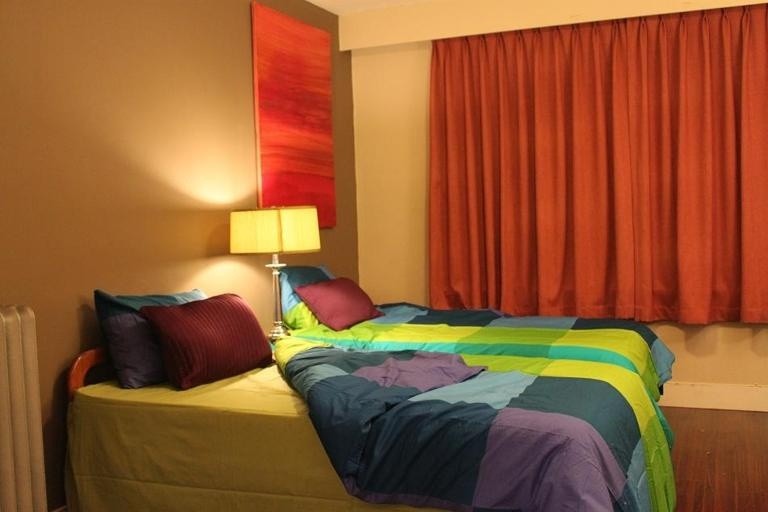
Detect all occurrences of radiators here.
[0,304,48,512]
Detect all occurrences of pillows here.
[138,292,277,389]
[94,288,209,389]
[294,276,386,331]
[278,265,337,332]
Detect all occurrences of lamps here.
[229,206,321,346]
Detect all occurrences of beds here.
[286,303,675,402]
[64,347,677,512]
